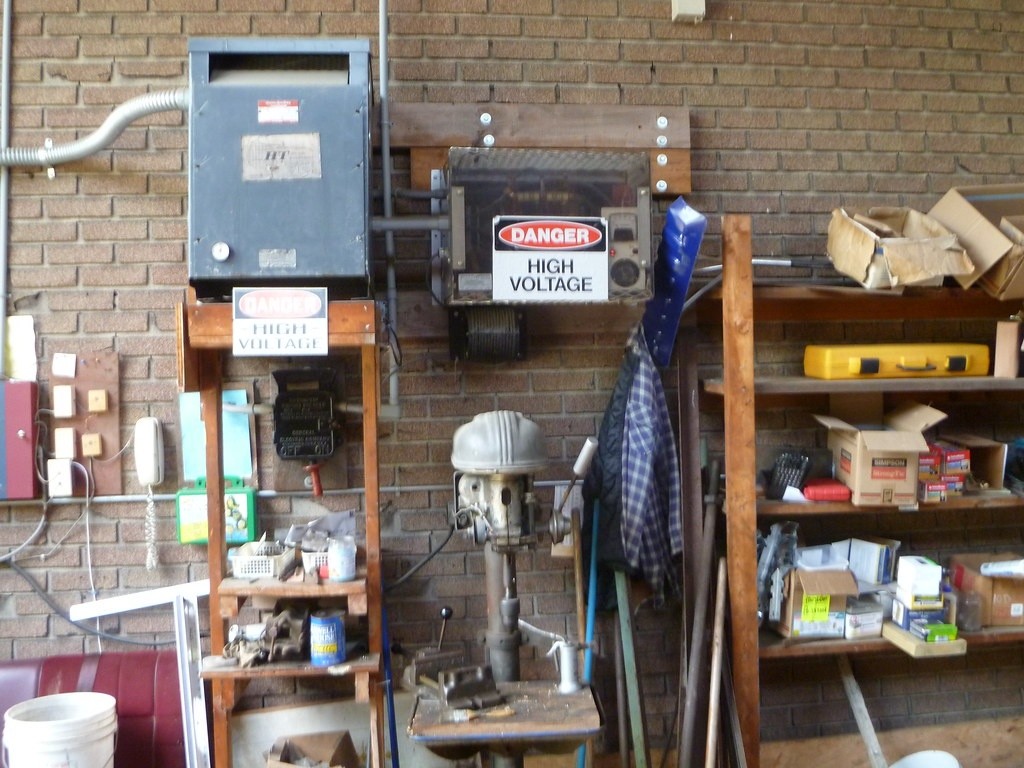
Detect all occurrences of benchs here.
[1,651,187,768]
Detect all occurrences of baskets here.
[300,547,329,575]
[229,540,295,579]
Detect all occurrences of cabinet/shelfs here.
[681,209,1024,768]
[177,285,387,768]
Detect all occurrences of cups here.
[329,537,356,581]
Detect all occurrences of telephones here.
[135,415,166,488]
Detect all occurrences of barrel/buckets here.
[1,692,118,768]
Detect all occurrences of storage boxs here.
[765,535,1023,657]
[809,391,948,506]
[267,729,359,768]
[827,181,1023,302]
